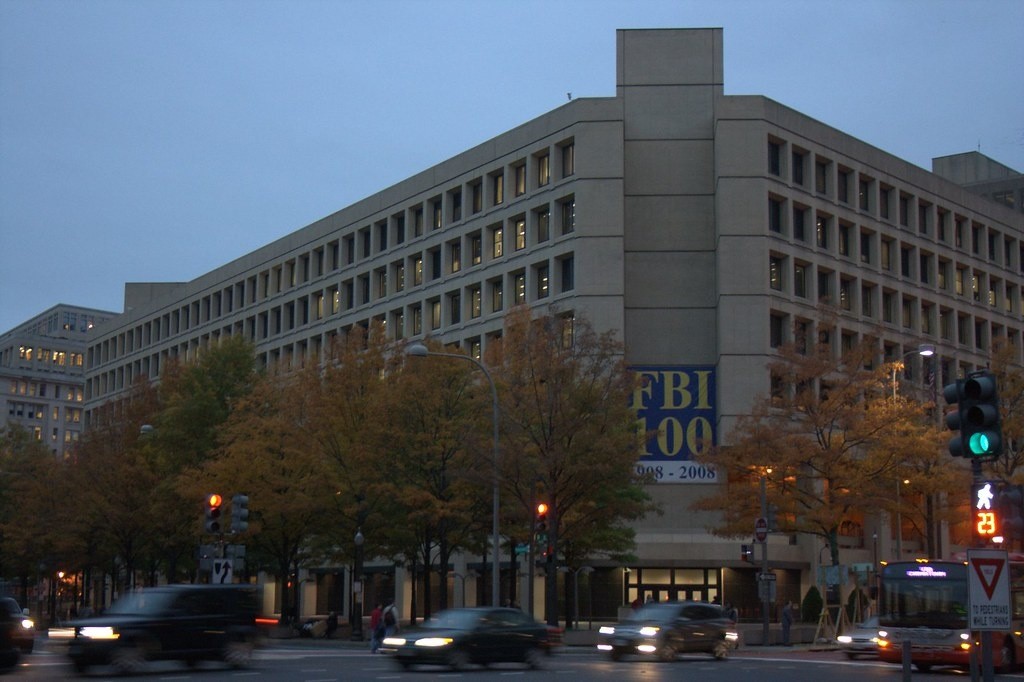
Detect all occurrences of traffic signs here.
[760,572,778,581]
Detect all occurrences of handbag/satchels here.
[384,606,396,627]
[375,621,385,639]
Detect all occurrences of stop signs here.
[754,519,766,540]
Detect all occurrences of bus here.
[869,560,1024,674]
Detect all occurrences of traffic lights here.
[942,370,1003,461]
[204,492,224,535]
[972,483,1001,539]
[533,501,551,533]
[230,494,249,534]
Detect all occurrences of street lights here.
[406,345,501,607]
[353,528,366,640]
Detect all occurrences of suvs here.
[597,601,741,663]
[47,585,259,674]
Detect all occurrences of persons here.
[645,594,654,605]
[369,602,383,654]
[322,611,338,639]
[630,595,644,609]
[780,600,794,647]
[726,603,739,649]
[381,598,400,635]
[711,596,720,605]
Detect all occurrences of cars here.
[0,597,35,655]
[377,606,565,673]
[836,615,880,660]
